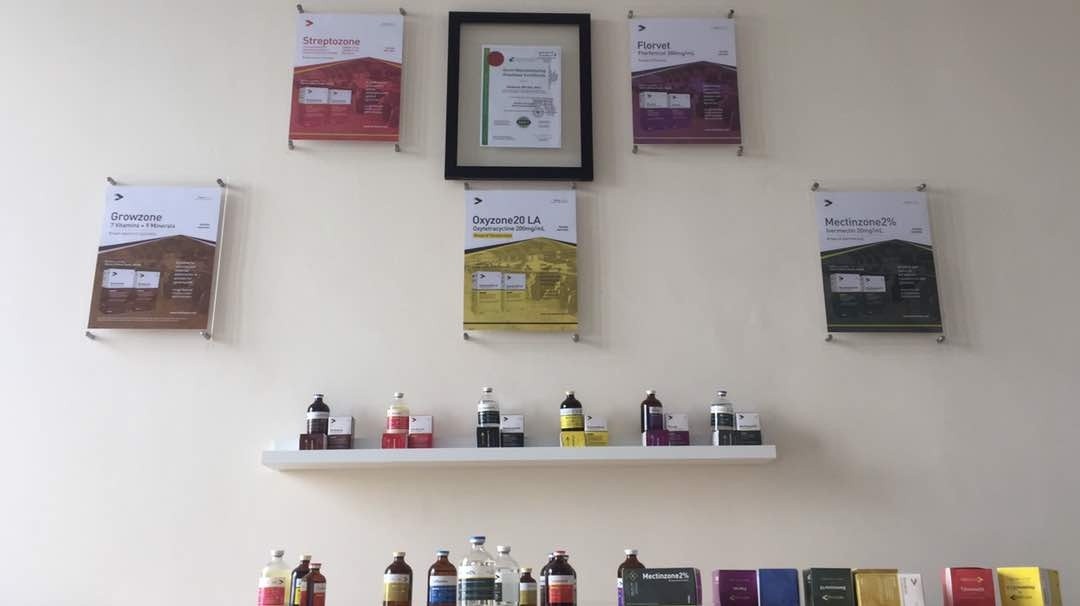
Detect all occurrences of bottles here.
[559,390,584,432]
[639,389,665,433]
[616,549,646,606]
[520,548,578,606]
[257,550,293,606]
[494,545,521,606]
[382,551,413,606]
[386,392,411,436]
[289,554,327,605]
[305,393,330,437]
[454,536,497,606]
[427,550,458,606]
[476,388,500,429]
[710,389,734,432]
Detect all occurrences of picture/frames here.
[445,11,594,181]
